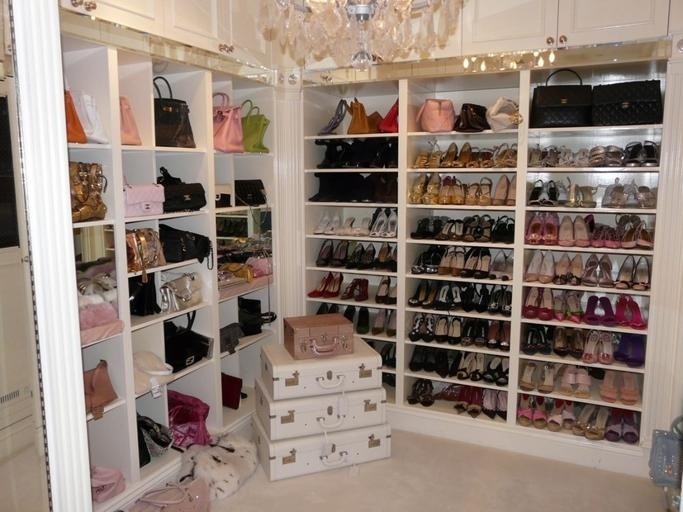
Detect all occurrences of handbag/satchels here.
[127,476,210,512]
[414,68,668,134]
[234,179,269,225]
[648,415,682,487]
[83,358,119,421]
[212,91,245,153]
[220,322,244,354]
[164,310,214,373]
[124,223,214,316]
[180,434,258,501]
[78,257,124,347]
[75,92,107,146]
[221,373,248,410]
[215,183,233,208]
[153,76,197,148]
[238,296,276,335]
[64,89,87,144]
[157,167,207,214]
[122,175,166,219]
[137,411,174,458]
[215,208,273,300]
[240,99,270,154]
[133,352,175,398]
[318,98,398,135]
[120,96,142,145]
[70,161,108,224]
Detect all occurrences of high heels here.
[315,303,395,337]
[409,173,656,203]
[414,140,659,170]
[410,246,652,293]
[408,280,646,332]
[411,212,653,250]
[313,208,397,240]
[309,272,397,306]
[316,239,397,272]
[367,341,395,387]
[407,379,640,446]
[408,346,640,408]
[408,314,646,369]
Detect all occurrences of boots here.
[314,138,399,169]
[309,172,397,204]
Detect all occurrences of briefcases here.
[283,313,355,361]
[254,377,387,443]
[252,413,392,483]
[260,335,383,402]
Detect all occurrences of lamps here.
[252,1,471,88]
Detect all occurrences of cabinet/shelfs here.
[461,1,671,56]
[54,0,157,37]
[297,27,680,484]
[50,5,280,512]
[156,1,274,69]
[300,3,461,72]
